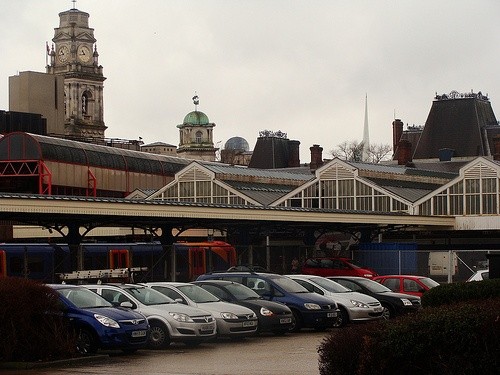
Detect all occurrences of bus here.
[1,239,238,288]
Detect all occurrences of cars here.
[144,282,259,340]
[281,275,384,329]
[71,280,218,350]
[467,269,490,283]
[372,274,440,297]
[189,280,295,334]
[19,282,150,358]
[326,275,423,319]
[298,258,376,280]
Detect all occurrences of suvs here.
[194,267,341,329]
